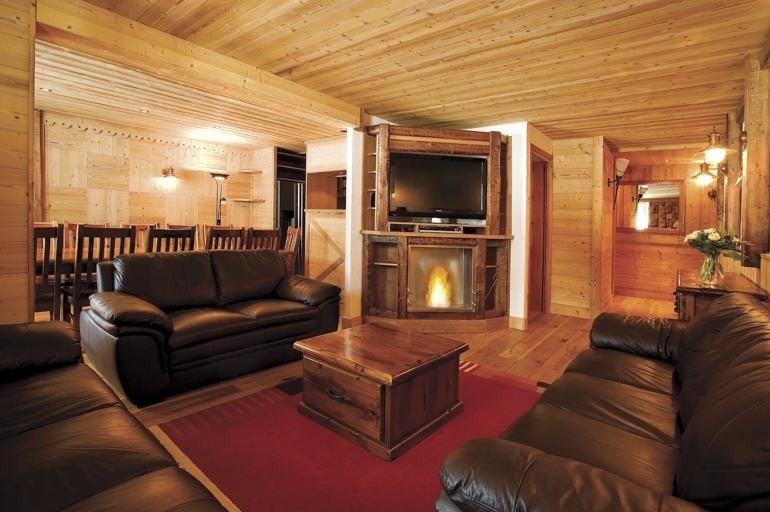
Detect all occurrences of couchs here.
[79,246,344,414]
[428,285,770,512]
[1,318,234,512]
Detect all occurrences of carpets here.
[146,358,545,512]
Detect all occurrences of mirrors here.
[612,177,687,236]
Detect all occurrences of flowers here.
[684,228,756,280]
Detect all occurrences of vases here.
[700,257,731,283]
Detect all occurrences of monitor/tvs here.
[389,152,487,224]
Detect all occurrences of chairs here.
[35,217,304,324]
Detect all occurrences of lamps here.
[693,126,750,166]
[630,182,648,213]
[607,157,630,212]
[691,161,728,186]
[154,166,183,194]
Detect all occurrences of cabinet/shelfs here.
[674,267,770,325]
[359,221,513,319]
